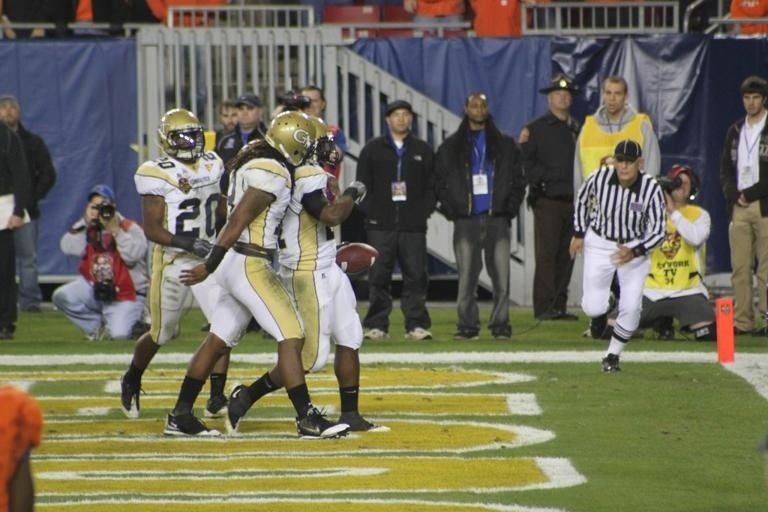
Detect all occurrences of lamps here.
[318,3,468,43]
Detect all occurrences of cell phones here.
[94,279,114,301]
[91,201,114,222]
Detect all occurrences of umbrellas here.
[537,311,580,321]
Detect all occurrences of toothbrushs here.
[335,243,379,275]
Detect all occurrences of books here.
[0,193,31,234]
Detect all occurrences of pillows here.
[309,116,331,160]
[264,110,317,168]
[158,107,204,162]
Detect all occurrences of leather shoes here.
[342,179,367,206]
[172,233,212,257]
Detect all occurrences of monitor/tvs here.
[0,193,31,234]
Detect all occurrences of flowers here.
[689,187,701,200]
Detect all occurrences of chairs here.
[85,324,108,341]
[733,326,768,338]
[204,397,230,418]
[403,327,432,341]
[363,328,390,340]
[453,330,479,341]
[224,381,249,434]
[119,373,148,419]
[589,290,617,339]
[163,410,223,438]
[294,408,351,439]
[599,356,622,374]
[653,317,676,341]
[494,333,511,340]
[336,414,391,432]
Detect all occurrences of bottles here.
[613,139,643,161]
[384,99,412,116]
[88,183,115,203]
[234,92,262,108]
[538,72,581,96]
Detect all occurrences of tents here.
[677,164,698,193]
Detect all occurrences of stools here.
[591,225,633,243]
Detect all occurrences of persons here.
[432,92,528,342]
[567,139,667,375]
[717,75,768,340]
[120,108,229,419]
[402,0,469,37]
[519,74,587,323]
[600,164,720,340]
[466,0,531,38]
[225,115,391,438]
[201,86,348,339]
[348,100,438,346]
[146,0,229,27]
[2,382,45,511]
[1,92,57,315]
[50,183,150,344]
[727,0,768,34]
[0,120,33,339]
[163,111,351,440]
[571,72,663,342]
